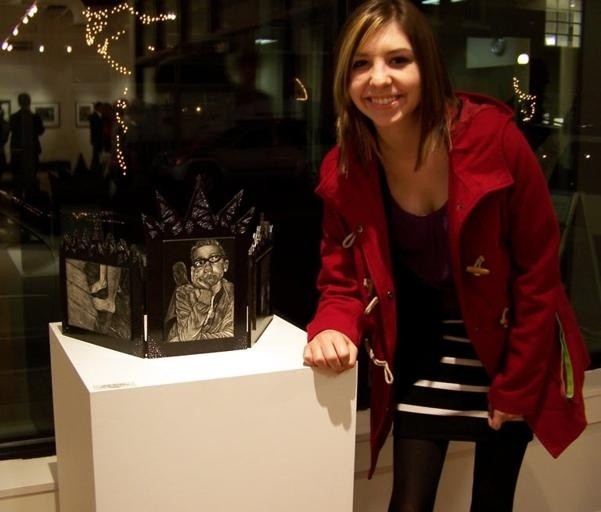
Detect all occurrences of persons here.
[6,93,46,203]
[86,101,324,330]
[87,264,122,314]
[165,238,237,343]
[303,1,596,511]
[0,108,11,177]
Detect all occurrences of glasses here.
[192,255,227,268]
